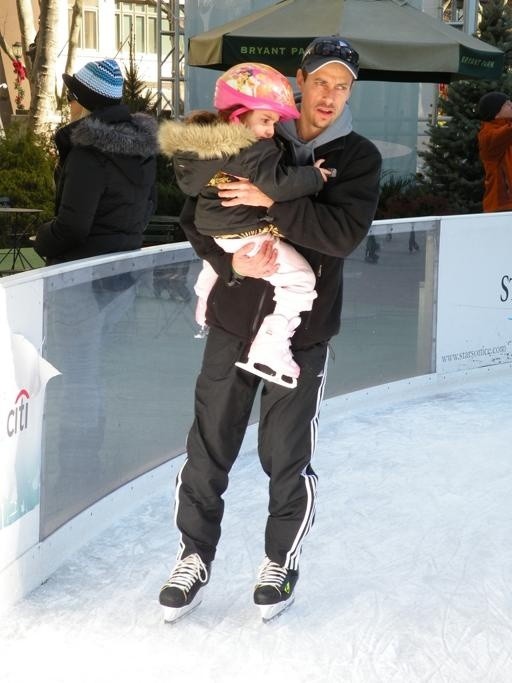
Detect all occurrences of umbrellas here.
[187,0,506,84]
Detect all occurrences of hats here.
[477,92,510,123]
[301,37,360,83]
[62,59,124,113]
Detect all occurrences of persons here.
[156,35,384,610]
[28,56,160,495]
[146,58,333,381]
[151,228,422,305]
[475,89,511,212]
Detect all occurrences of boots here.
[249,313,301,379]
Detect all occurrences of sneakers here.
[254,561,299,605]
[196,297,207,328]
[159,548,211,608]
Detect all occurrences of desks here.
[1,207,46,273]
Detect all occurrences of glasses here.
[67,92,77,102]
[312,44,360,64]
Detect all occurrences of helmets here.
[214,62,301,122]
